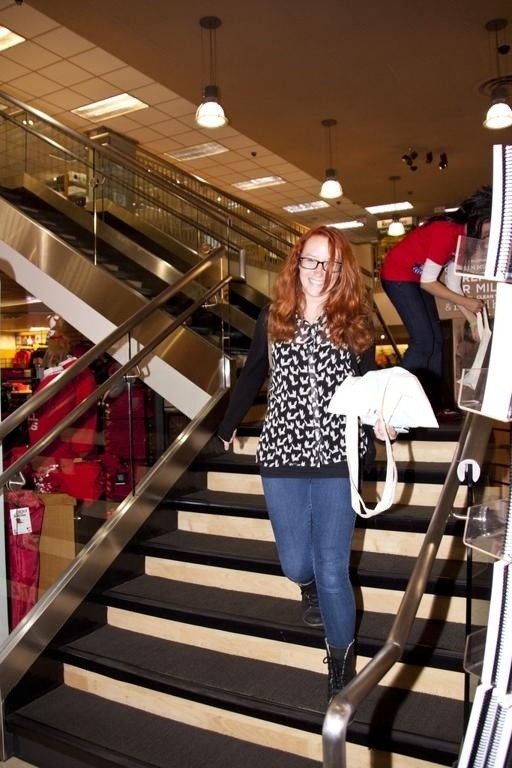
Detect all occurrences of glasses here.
[298,257,341,273]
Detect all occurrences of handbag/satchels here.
[327,367,440,430]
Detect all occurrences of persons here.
[217,227,398,728]
[379,185,492,416]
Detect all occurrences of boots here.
[325,637,354,726]
[301,577,322,626]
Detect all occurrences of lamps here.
[399,149,420,172]
[386,170,405,238]
[477,12,512,137]
[434,151,450,174]
[426,148,434,166]
[194,11,229,133]
[317,112,348,205]
